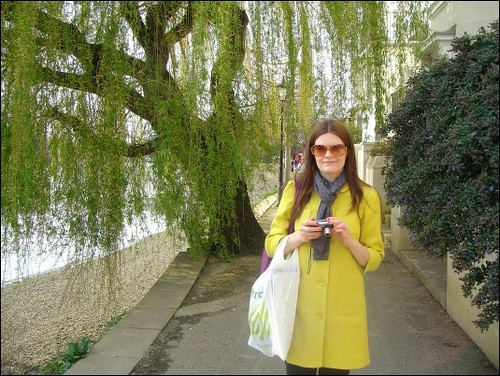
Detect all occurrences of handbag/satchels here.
[260,179,301,276]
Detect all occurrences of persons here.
[263,119,385,376]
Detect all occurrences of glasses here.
[310,144,348,156]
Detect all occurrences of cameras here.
[315,219,333,237]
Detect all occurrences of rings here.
[342,224,349,230]
